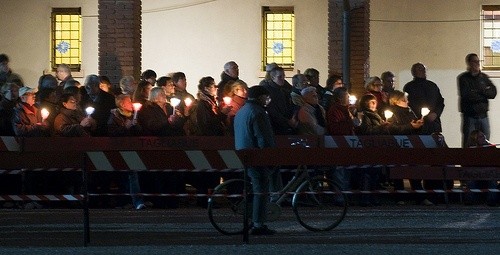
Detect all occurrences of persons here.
[234,85,278,235]
[0,54,431,208]
[459,130,496,148]
[426,132,456,191]
[457,54,497,148]
[403,63,445,136]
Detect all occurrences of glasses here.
[372,83,383,86]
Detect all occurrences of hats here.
[19,86,36,97]
[266,62,277,71]
[247,85,270,98]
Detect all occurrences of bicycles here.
[208,137,349,235]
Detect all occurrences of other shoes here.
[336,198,500,207]
[251,224,277,235]
[133,199,153,210]
